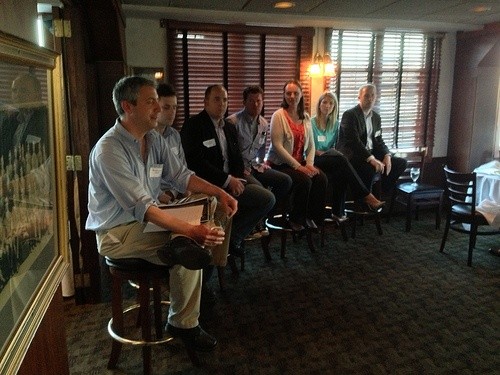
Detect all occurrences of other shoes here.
[362,197,387,211]
[256,221,269,237]
[288,221,311,236]
[331,214,349,226]
[165,322,218,352]
[305,218,321,234]
[247,226,262,239]
[157,237,212,270]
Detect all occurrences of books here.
[141,197,209,234]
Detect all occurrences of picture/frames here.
[0,31,71,375]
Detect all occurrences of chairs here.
[440,165,500,266]
[386,150,445,233]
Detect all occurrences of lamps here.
[306,27,337,78]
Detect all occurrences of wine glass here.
[410,167,420,187]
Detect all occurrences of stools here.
[216,173,383,293]
[105,256,200,375]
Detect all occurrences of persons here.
[0,73,49,214]
[84,76,238,347]
[225,86,293,238]
[269,79,327,233]
[337,83,406,214]
[303,91,387,228]
[152,83,232,283]
[179,84,275,258]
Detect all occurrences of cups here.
[210,217,226,231]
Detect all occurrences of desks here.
[461,159,500,231]
